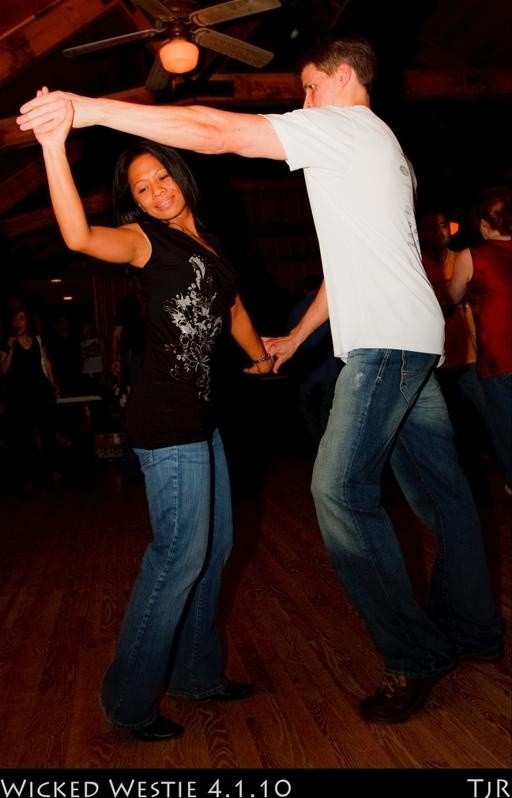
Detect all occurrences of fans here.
[64,2,284,92]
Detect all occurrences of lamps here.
[158,36,202,75]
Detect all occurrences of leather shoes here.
[213,679,255,700]
[137,718,183,740]
[359,654,456,722]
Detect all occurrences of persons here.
[15,40,510,724]
[30,77,283,740]
[1,184,510,495]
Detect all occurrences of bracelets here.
[251,354,273,364]
